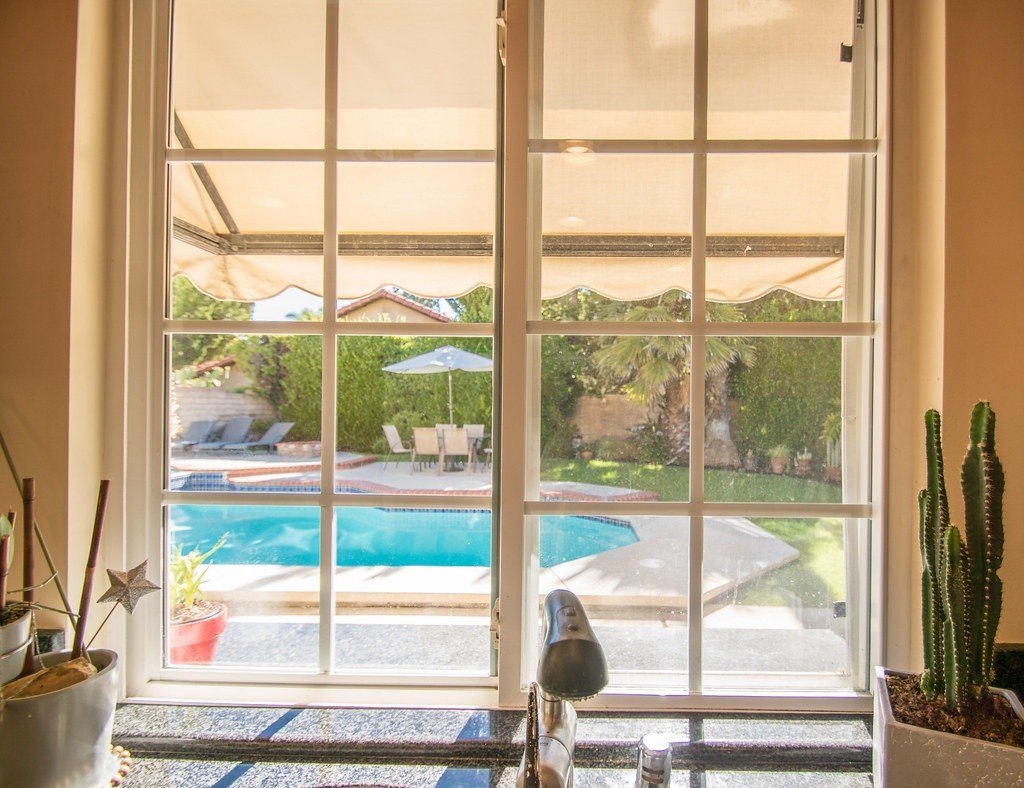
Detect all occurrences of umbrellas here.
[381,344,493,424]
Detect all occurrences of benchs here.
[222,422,295,453]
[381,423,427,474]
[190,417,254,452]
[170,419,214,449]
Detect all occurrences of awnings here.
[161,0,857,307]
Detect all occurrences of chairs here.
[410,422,493,475]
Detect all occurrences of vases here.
[1,649,120,788]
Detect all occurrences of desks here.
[409,432,490,473]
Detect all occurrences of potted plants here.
[872,397,1023,787]
[766,444,792,475]
[578,444,594,460]
[1,514,33,685]
[796,446,812,466]
[170,531,229,665]
